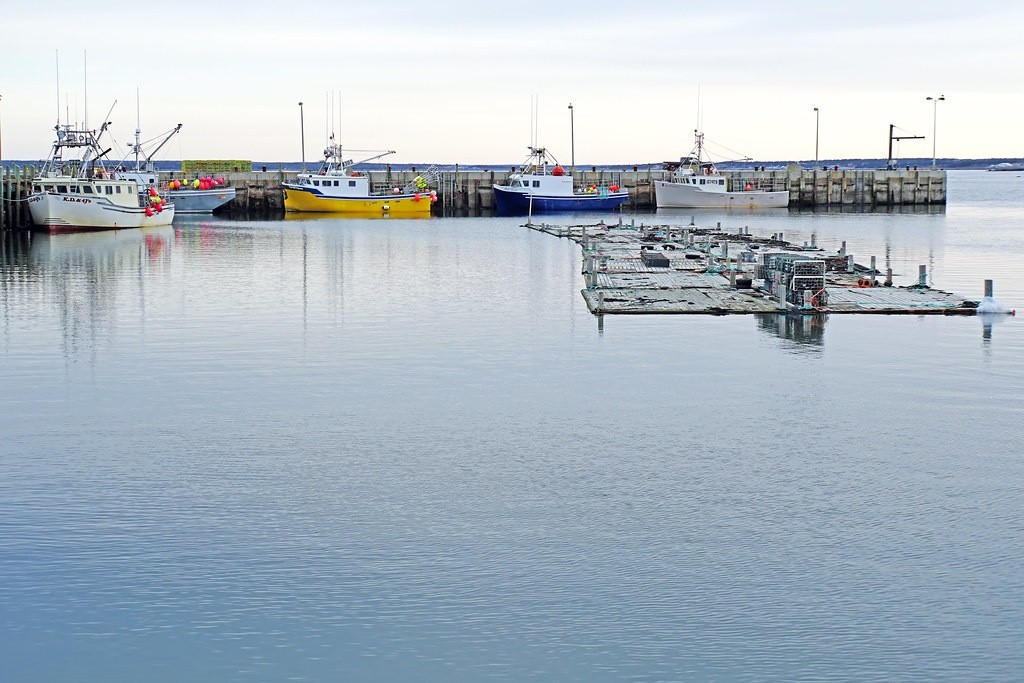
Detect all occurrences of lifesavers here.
[705,169,709,174]
[863,280,871,288]
[56,129,66,138]
[670,164,675,171]
[78,134,85,142]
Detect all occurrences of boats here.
[281,91,437,212]
[655,85,789,209]
[115,84,237,215]
[987,163,1024,171]
[283,210,431,335]
[491,92,630,218]
[26,50,183,232]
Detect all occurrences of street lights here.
[814,108,819,164]
[926,96,945,165]
[568,103,575,168]
[298,100,305,170]
